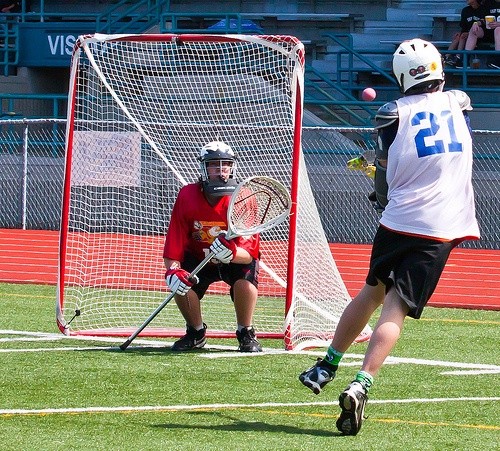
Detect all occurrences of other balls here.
[361,88,376,102]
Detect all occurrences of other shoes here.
[441,56,448,64]
[446,56,461,66]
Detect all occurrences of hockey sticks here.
[118,176,293,352]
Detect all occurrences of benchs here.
[165,13,500,99]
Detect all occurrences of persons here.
[298,38,480,437]
[162,141,261,352]
[443,0,500,69]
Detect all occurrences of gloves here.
[165,267,200,296]
[209,233,237,264]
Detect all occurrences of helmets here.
[197,141,238,196]
[392,38,445,94]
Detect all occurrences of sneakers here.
[171,323,207,351]
[454,60,471,69]
[236,326,262,352]
[298,357,335,394]
[336,380,369,436]
[488,58,500,69]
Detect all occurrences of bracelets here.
[474,22,477,24]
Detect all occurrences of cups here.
[485,16,496,29]
[472,59,480,69]
